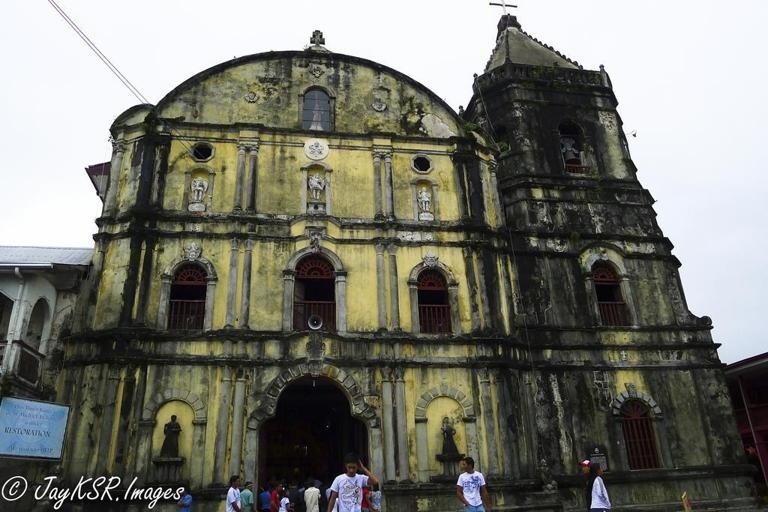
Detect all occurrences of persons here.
[159,415,182,459]
[561,138,580,171]
[578,143,596,171]
[178,490,194,512]
[588,463,614,512]
[440,417,461,456]
[228,450,383,512]
[455,457,492,512]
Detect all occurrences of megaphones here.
[308,314,323,330]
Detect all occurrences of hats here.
[578,459,593,469]
